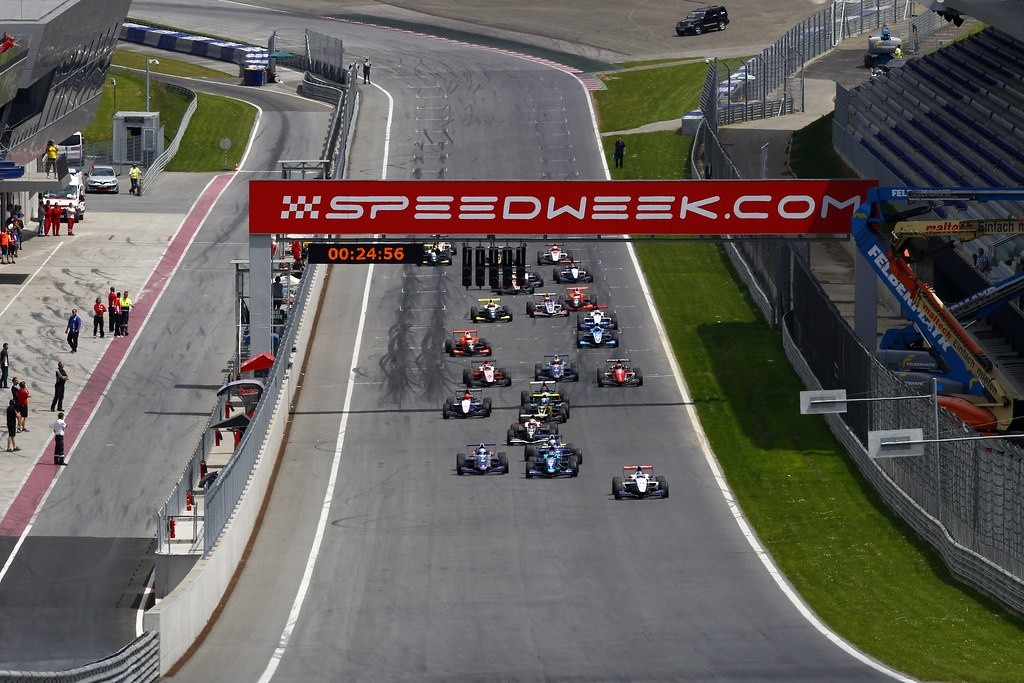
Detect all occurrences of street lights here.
[110,77,117,115]
[146,57,159,112]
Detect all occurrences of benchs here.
[847,28,1024,311]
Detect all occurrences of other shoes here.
[129,190,132,195]
[58,409,65,411]
[133,191,135,194]
[54,177,58,179]
[8,262,11,264]
[7,449,13,452]
[51,409,54,411]
[71,349,75,353]
[4,385,9,388]
[12,261,16,263]
[47,177,50,178]
[56,234,59,236]
[23,429,29,432]
[13,447,21,451]
[17,429,22,433]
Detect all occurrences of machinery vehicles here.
[854,186,1023,433]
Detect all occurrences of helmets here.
[49,140,54,143]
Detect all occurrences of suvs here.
[675,5,730,36]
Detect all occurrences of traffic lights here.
[515,246,526,286]
[475,246,486,286]
[488,246,499,287]
[502,249,514,289]
[461,247,472,286]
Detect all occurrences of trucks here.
[39,167,85,223]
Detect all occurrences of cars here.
[84,164,121,194]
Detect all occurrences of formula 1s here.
[612,465,669,500]
[471,298,513,323]
[416,235,458,266]
[507,414,559,447]
[535,353,579,383]
[518,381,568,404]
[597,358,643,387]
[518,394,568,424]
[537,243,573,266]
[485,245,543,295]
[525,286,620,349]
[524,434,575,461]
[463,359,511,388]
[553,260,594,284]
[526,448,579,479]
[443,388,492,419]
[445,329,492,357]
[456,441,509,473]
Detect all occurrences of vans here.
[53,130,86,166]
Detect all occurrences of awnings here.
[210,412,252,429]
[217,378,264,396]
[241,352,274,371]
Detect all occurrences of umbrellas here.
[269,50,295,82]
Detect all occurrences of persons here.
[93,298,107,338]
[45,140,60,179]
[895,45,904,59]
[614,136,626,169]
[363,57,372,84]
[38,200,62,237]
[65,309,81,354]
[349,59,360,80]
[11,376,31,433]
[271,276,284,310]
[0,204,24,265]
[0,32,19,54]
[7,400,21,452]
[66,203,76,236]
[53,413,68,465]
[129,164,142,195]
[108,287,134,336]
[0,343,9,388]
[51,362,69,412]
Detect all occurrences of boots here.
[54,455,59,465]
[59,456,68,465]
